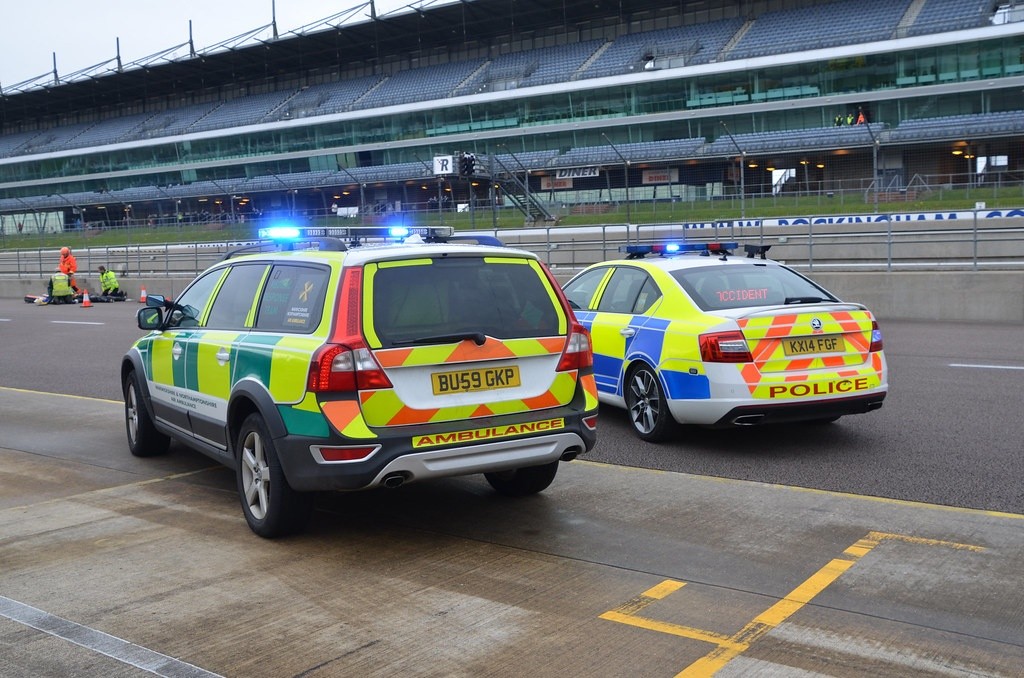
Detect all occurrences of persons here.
[98,266,127,296]
[49,247,127,305]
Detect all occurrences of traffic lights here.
[458,155,475,178]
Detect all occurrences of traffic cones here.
[138,282,148,303]
[79,288,94,308]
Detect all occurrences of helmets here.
[61,247,68,254]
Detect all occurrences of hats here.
[55,268,61,272]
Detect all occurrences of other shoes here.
[54,297,59,304]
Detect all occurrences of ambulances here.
[120,224,598,540]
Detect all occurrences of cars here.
[559,244,888,444]
